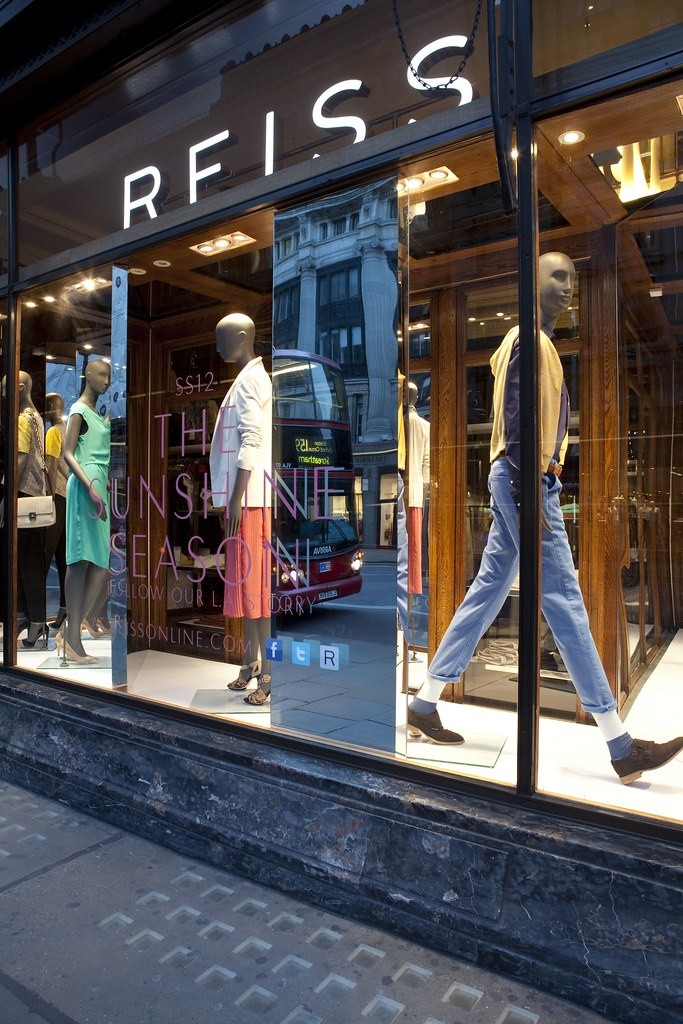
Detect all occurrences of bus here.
[163,349,361,616]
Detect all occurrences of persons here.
[0,371,50,648]
[208,313,271,705]
[408,252,683,787]
[397,370,431,642]
[55,359,111,665]
[45,392,73,629]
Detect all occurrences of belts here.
[500,449,563,475]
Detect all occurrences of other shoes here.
[407,706,465,745]
[611,737,683,784]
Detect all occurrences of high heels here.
[83,617,102,637]
[22,623,49,647]
[243,673,271,705]
[97,618,111,633]
[16,619,30,639]
[228,661,262,690]
[61,638,99,664]
[48,612,67,629]
[55,632,63,656]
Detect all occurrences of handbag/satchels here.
[17,496,56,528]
